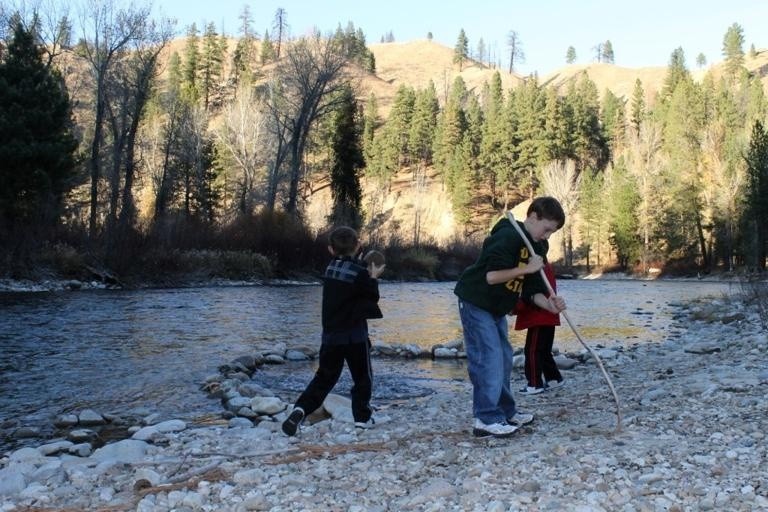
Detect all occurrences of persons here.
[281,226,388,438]
[452,196,570,437]
[504,235,567,395]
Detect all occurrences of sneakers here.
[281,406,306,436]
[354,417,376,429]
[515,384,545,396]
[473,411,535,438]
[544,375,565,391]
